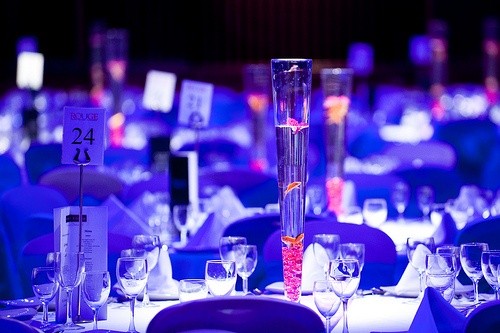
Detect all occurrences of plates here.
[0,298,41,319]
[265,282,330,295]
[380,285,475,297]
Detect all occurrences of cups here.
[178,279,207,303]
[489,254,500,279]
[45,252,61,284]
[425,275,455,305]
[305,186,388,227]
[497,264,500,288]
[436,246,462,278]
[205,260,236,296]
[426,254,457,276]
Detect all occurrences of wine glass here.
[393,184,433,229]
[55,251,85,331]
[460,242,489,305]
[218,237,247,294]
[313,234,339,280]
[340,244,365,298]
[314,280,343,333]
[233,246,257,294]
[403,237,437,304]
[116,258,147,333]
[329,259,360,333]
[33,267,59,331]
[82,272,111,333]
[130,235,161,308]
[481,250,500,300]
